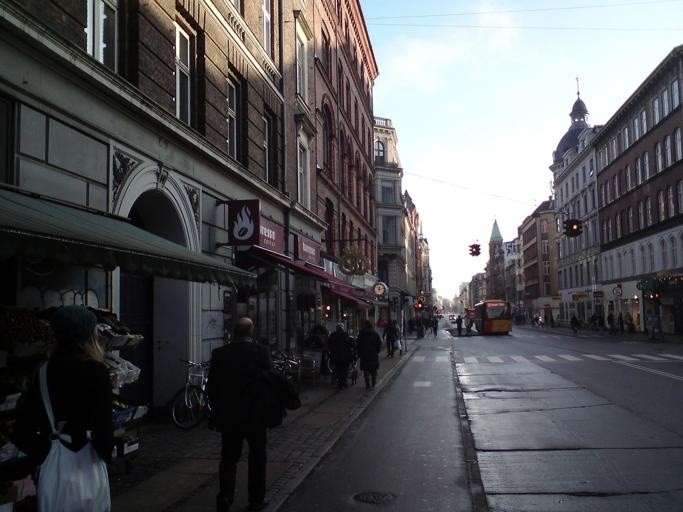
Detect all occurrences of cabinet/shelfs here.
[85,301,149,468]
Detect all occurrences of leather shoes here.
[216,501,231,511]
[250,497,269,509]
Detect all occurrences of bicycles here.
[272,346,301,387]
[170,351,215,432]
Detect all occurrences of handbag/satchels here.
[36,433,110,511]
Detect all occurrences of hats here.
[49,304,98,342]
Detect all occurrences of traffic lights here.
[468,243,474,257]
[562,218,584,236]
[412,303,425,309]
[475,244,480,256]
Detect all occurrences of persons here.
[569,310,642,337]
[383,319,401,358]
[326,322,355,389]
[207,317,287,511]
[407,314,438,337]
[456,315,472,337]
[10,304,114,512]
[356,319,381,390]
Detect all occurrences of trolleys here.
[329,348,359,384]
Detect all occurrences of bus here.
[464,309,475,323]
[474,299,513,335]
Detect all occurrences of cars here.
[433,312,462,324]
